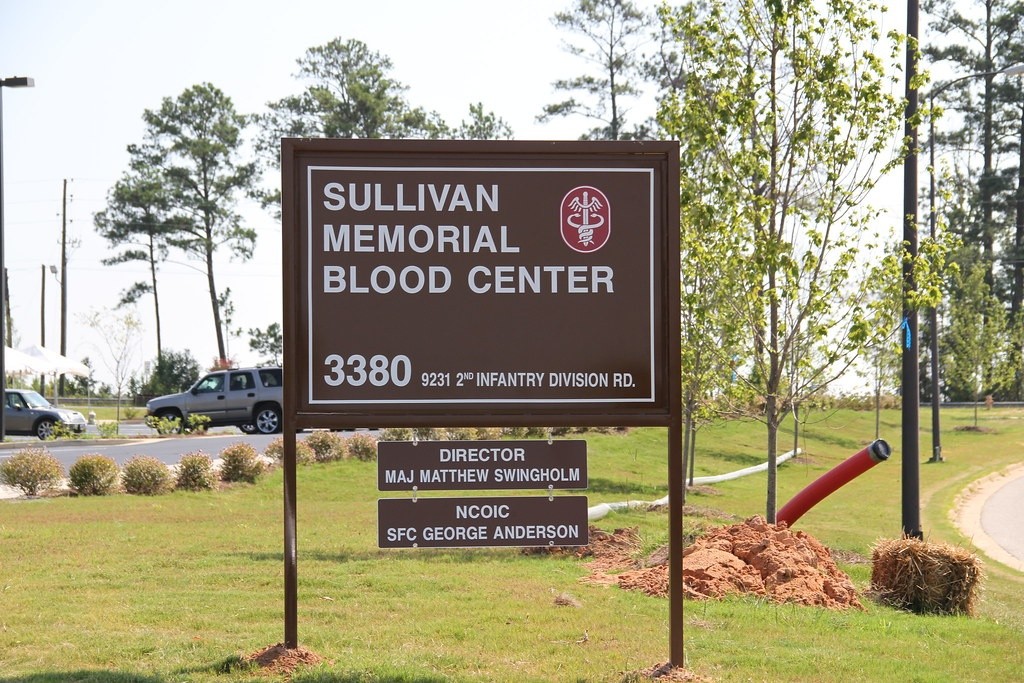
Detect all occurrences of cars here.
[5,389,87,441]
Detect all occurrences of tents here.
[4,345,92,408]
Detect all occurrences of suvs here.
[145,366,284,435]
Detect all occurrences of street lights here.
[51,265,67,397]
[926,61,1024,458]
[0,77,37,440]
